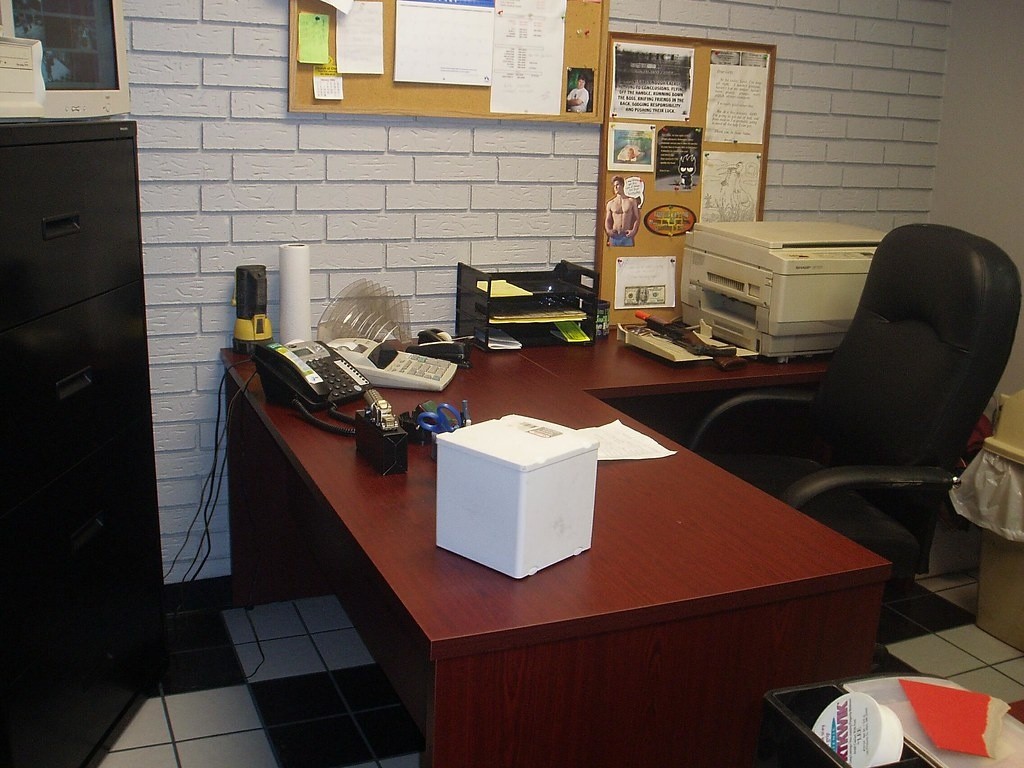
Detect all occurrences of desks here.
[218,329,894,768]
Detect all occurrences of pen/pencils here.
[462,399,471,428]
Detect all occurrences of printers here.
[680,222,886,362]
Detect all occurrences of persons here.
[567,74,589,112]
[624,148,637,163]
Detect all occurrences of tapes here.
[812,689,904,768]
[437,332,453,341]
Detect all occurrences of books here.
[550,322,590,342]
[475,328,522,349]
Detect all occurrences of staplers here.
[406,341,473,369]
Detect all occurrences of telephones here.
[252,339,375,415]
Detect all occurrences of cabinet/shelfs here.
[1,120,166,767]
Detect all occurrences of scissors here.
[417,404,461,434]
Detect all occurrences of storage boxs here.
[763,682,948,768]
[435,411,599,579]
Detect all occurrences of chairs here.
[693,223,1018,659]
